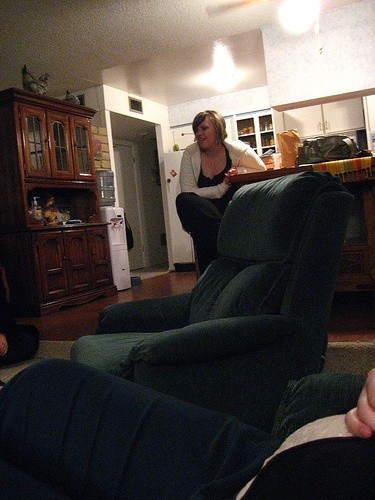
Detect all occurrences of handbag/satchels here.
[276,128,302,169]
[297,135,359,166]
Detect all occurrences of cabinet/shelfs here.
[234,108,285,155]
[0,87,117,319]
[282,97,365,140]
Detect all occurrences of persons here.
[0,359,375,500]
[0,279,39,368]
[176,110,267,276]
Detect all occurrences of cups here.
[270,153,282,170]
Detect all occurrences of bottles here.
[95,170,116,207]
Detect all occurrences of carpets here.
[0,340,375,417]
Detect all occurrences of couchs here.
[70,171,353,427]
